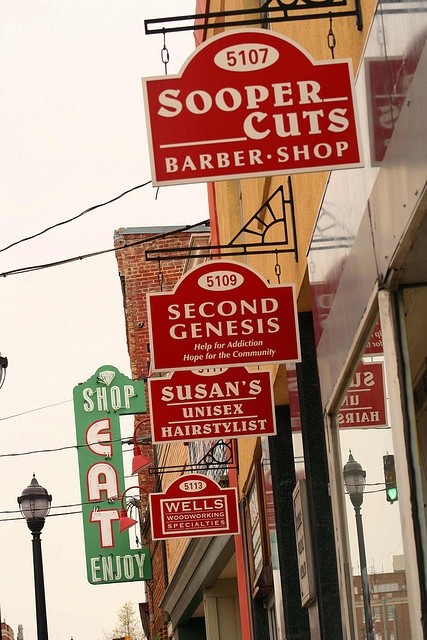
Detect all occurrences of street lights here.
[16,472,52,640]
[0,351,7,390]
[342,449,374,639]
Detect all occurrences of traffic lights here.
[382,455,397,505]
